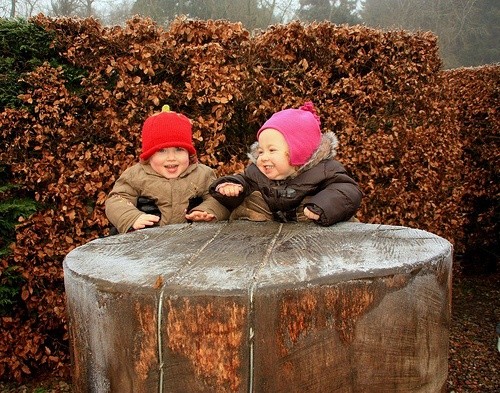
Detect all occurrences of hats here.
[139,111,197,163]
[257,102,322,166]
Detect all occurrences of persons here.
[105,105,232,234]
[207,102,362,227]
